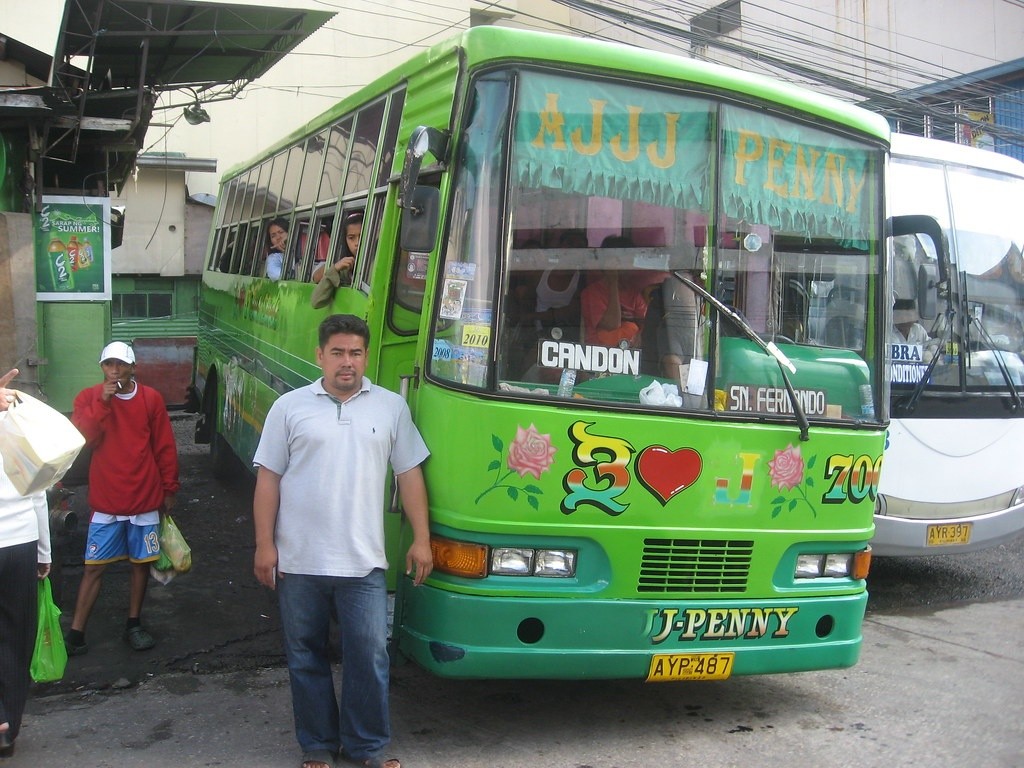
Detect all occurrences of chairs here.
[250,223,354,286]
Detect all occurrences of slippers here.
[341,748,401,768]
[123,626,155,650]
[63,639,88,656]
[301,749,336,768]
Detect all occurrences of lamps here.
[153,85,210,125]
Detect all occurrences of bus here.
[723,133,1024,559]
[184,21,949,682]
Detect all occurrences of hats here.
[98,341,135,364]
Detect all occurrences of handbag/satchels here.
[149,514,192,585]
[0,391,86,497]
[30,577,68,682]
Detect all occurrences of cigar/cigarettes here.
[117,381,122,388]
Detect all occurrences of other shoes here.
[0,734,14,758]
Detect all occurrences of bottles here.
[557,368,577,399]
[48,231,94,290]
[859,384,876,420]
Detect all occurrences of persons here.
[265,217,298,282]
[0,368,51,755]
[253,313,433,768]
[893,299,931,351]
[513,229,777,389]
[312,211,379,309]
[64,341,179,653]
